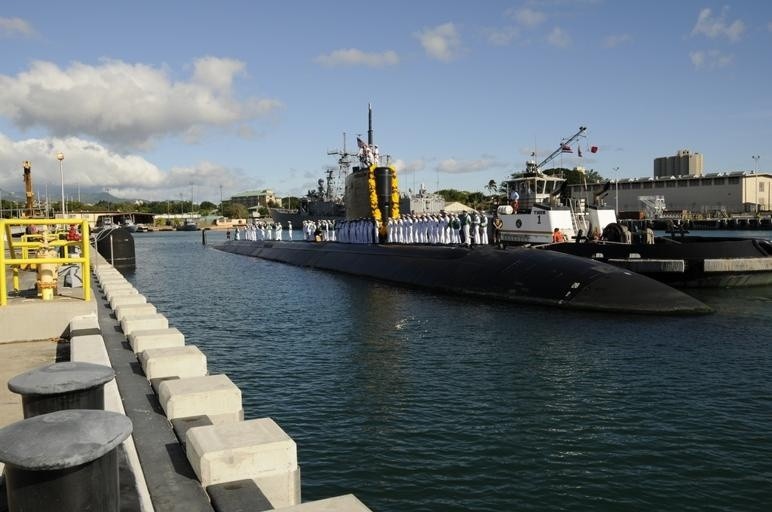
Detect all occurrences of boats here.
[94,212,205,232]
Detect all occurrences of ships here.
[214,99,771,319]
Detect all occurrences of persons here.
[591,225,603,241]
[510,186,521,216]
[223,209,489,247]
[551,227,565,245]
[356,144,379,168]
[491,210,504,245]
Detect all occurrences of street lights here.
[751,154,761,215]
[611,165,621,219]
[58,151,66,229]
[220,185,224,215]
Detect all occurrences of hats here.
[481,210,485,214]
[473,210,478,214]
[387,211,458,220]
[340,217,371,222]
[462,209,468,212]
